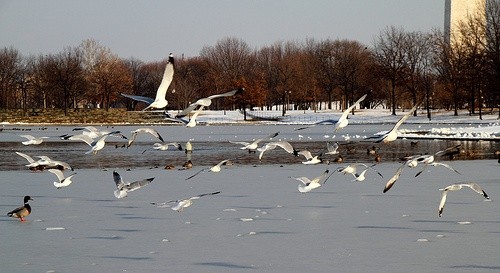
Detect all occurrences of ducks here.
[7,196,34,221]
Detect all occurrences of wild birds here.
[15,51,491,217]
